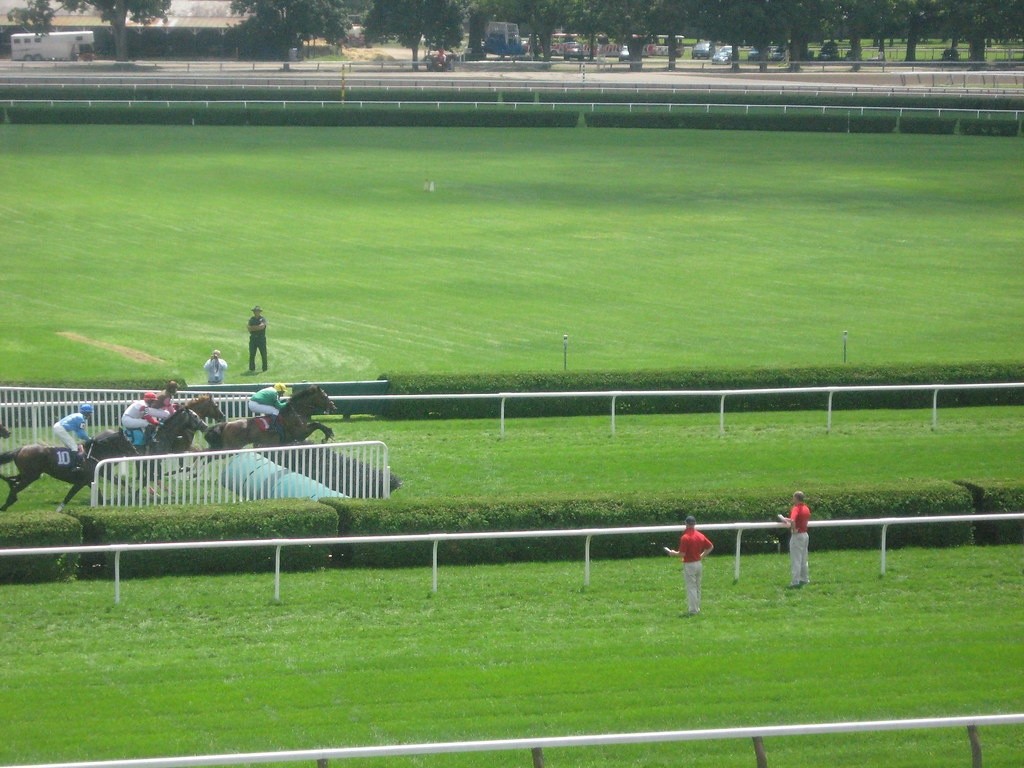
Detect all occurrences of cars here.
[618,46,629,61]
[819,49,839,59]
[845,50,862,62]
[691,41,715,60]
[712,46,741,64]
[941,49,960,61]
[747,47,760,60]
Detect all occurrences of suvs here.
[561,43,584,61]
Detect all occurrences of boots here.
[69,451,83,472]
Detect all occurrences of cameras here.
[213,355,217,359]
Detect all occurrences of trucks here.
[341,15,372,49]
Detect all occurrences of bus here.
[528,33,596,56]
[598,34,685,57]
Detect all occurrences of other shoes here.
[680,612,694,618]
[786,584,800,589]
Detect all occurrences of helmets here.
[80,404,93,413]
[165,381,179,392]
[144,392,158,400]
[273,382,287,391]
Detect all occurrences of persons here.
[667,516,714,613]
[205,350,227,385]
[53,405,93,471]
[121,381,178,446]
[783,491,810,587]
[248,306,267,371]
[248,383,288,430]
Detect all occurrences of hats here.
[251,306,263,311]
[684,516,695,524]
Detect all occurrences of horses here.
[1,384,337,515]
